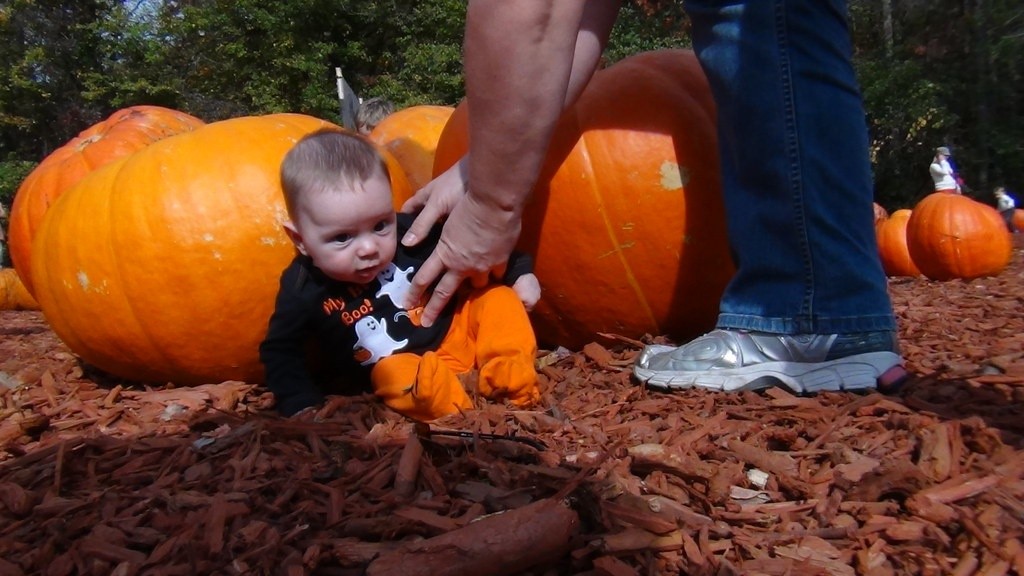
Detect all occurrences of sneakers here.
[632,326,907,393]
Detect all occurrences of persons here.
[993,186,1015,212]
[256,128,542,420]
[929,147,964,195]
[353,98,397,137]
[401,0,906,396]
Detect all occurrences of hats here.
[937,147,951,156]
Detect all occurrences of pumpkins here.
[1,50,1024,386]
